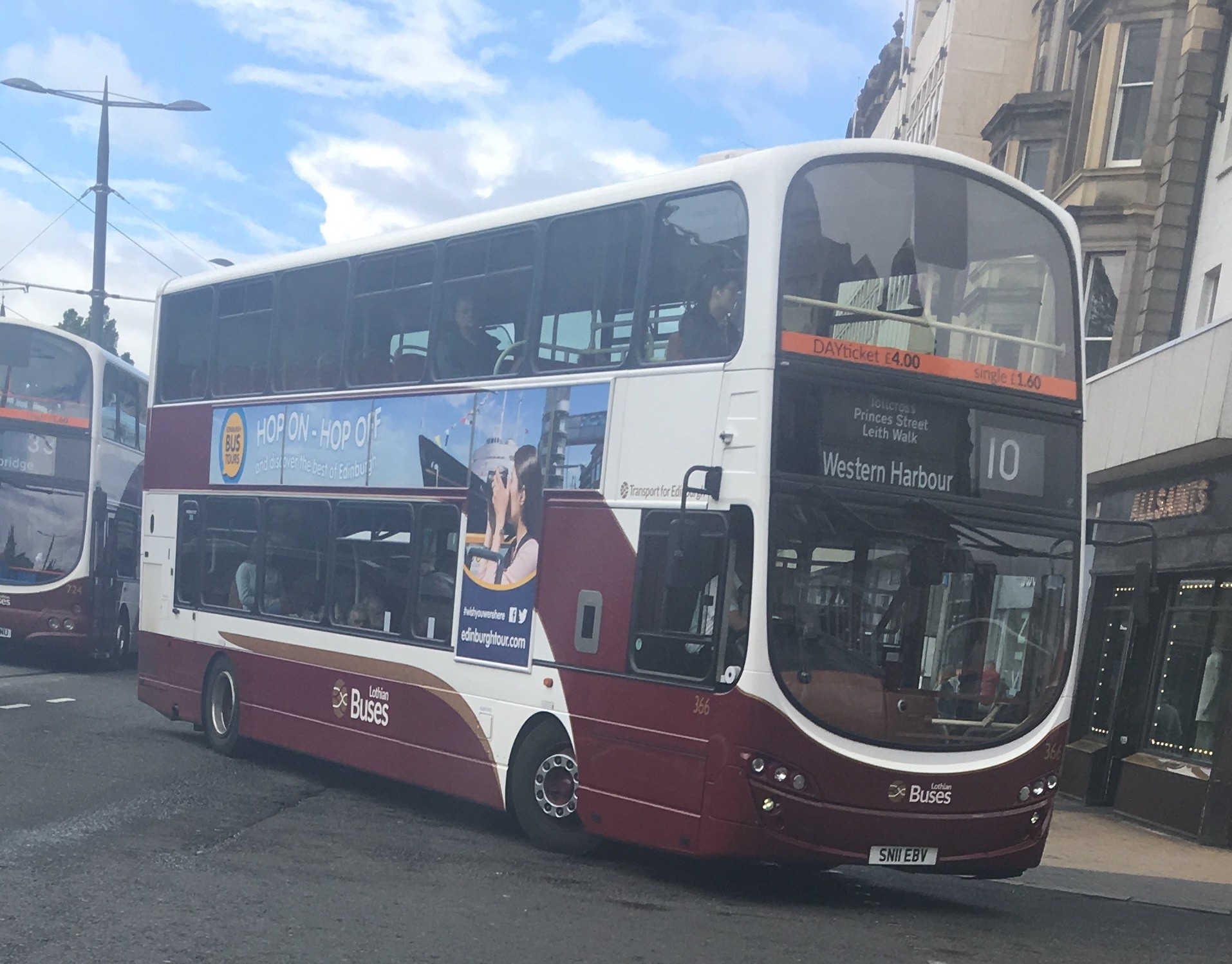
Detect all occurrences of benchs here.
[160,336,578,632]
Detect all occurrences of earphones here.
[717,291,721,296]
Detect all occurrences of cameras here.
[485,467,509,489]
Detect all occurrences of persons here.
[233,531,456,640]
[1158,700,1183,742]
[469,445,540,584]
[1192,645,1224,761]
[933,659,1002,718]
[683,557,749,656]
[435,299,506,379]
[666,275,741,358]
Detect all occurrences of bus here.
[135,138,1164,880]
[0,316,149,672]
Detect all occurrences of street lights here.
[0,72,215,349]
